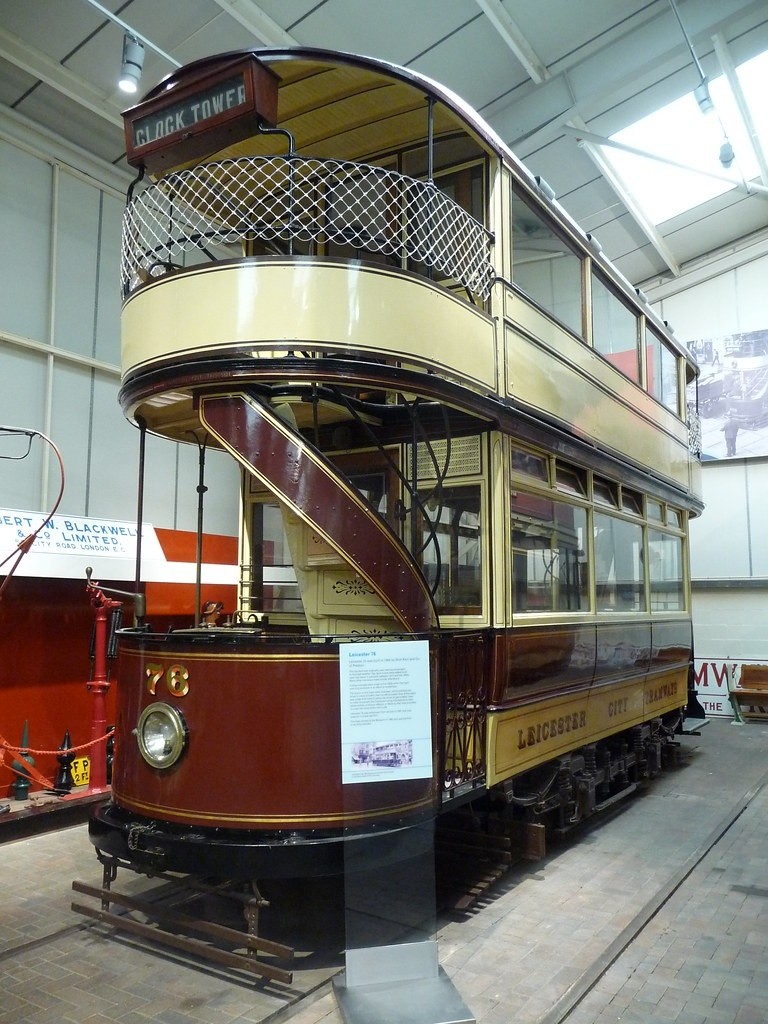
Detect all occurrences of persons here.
[711,348,721,366]
[720,414,739,458]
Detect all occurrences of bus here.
[67,44,713,980]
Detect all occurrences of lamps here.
[719,142,735,169]
[118,31,146,94]
[694,84,715,116]
[577,137,587,148]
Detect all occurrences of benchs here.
[727,663,768,725]
